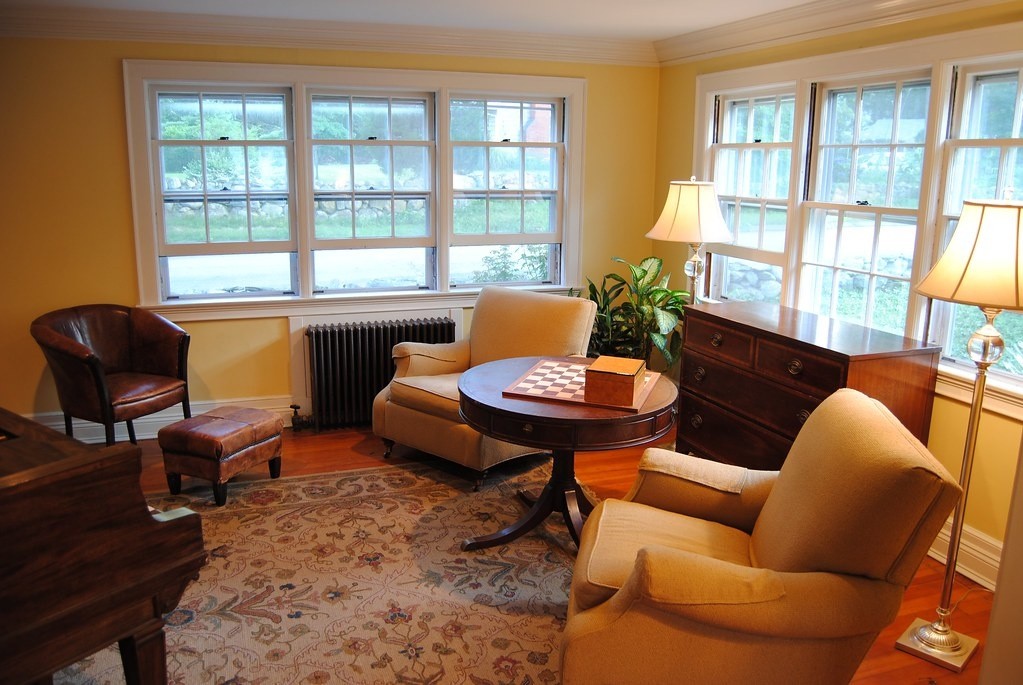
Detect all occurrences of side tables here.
[453,352,680,558]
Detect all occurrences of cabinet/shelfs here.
[674,295,946,472]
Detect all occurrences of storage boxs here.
[581,355,650,408]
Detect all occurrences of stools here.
[156,404,289,508]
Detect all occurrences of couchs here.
[548,383,971,685]
[29,301,193,446]
[365,284,601,493]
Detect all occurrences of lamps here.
[893,195,1023,671]
[640,171,741,304]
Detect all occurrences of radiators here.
[300,315,459,436]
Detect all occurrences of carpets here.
[43,449,605,685]
